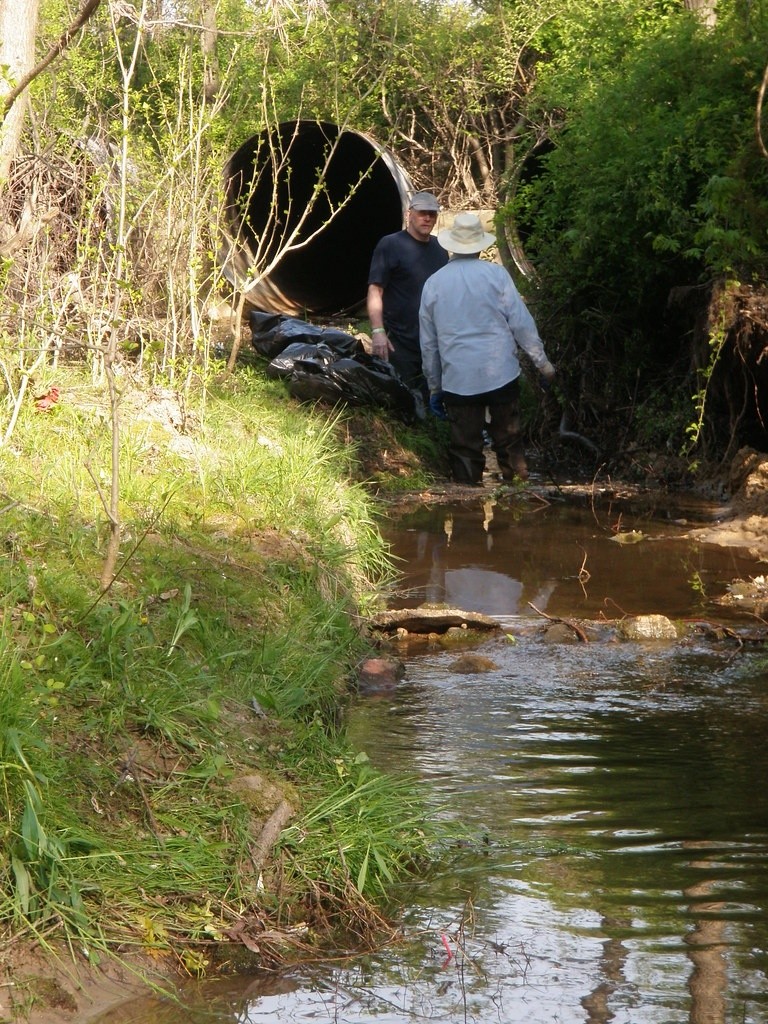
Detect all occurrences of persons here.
[426,499,562,616]
[366,191,450,389]
[419,210,555,483]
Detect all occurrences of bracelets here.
[370,327,386,334]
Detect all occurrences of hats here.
[438,214,497,253]
[409,192,440,212]
